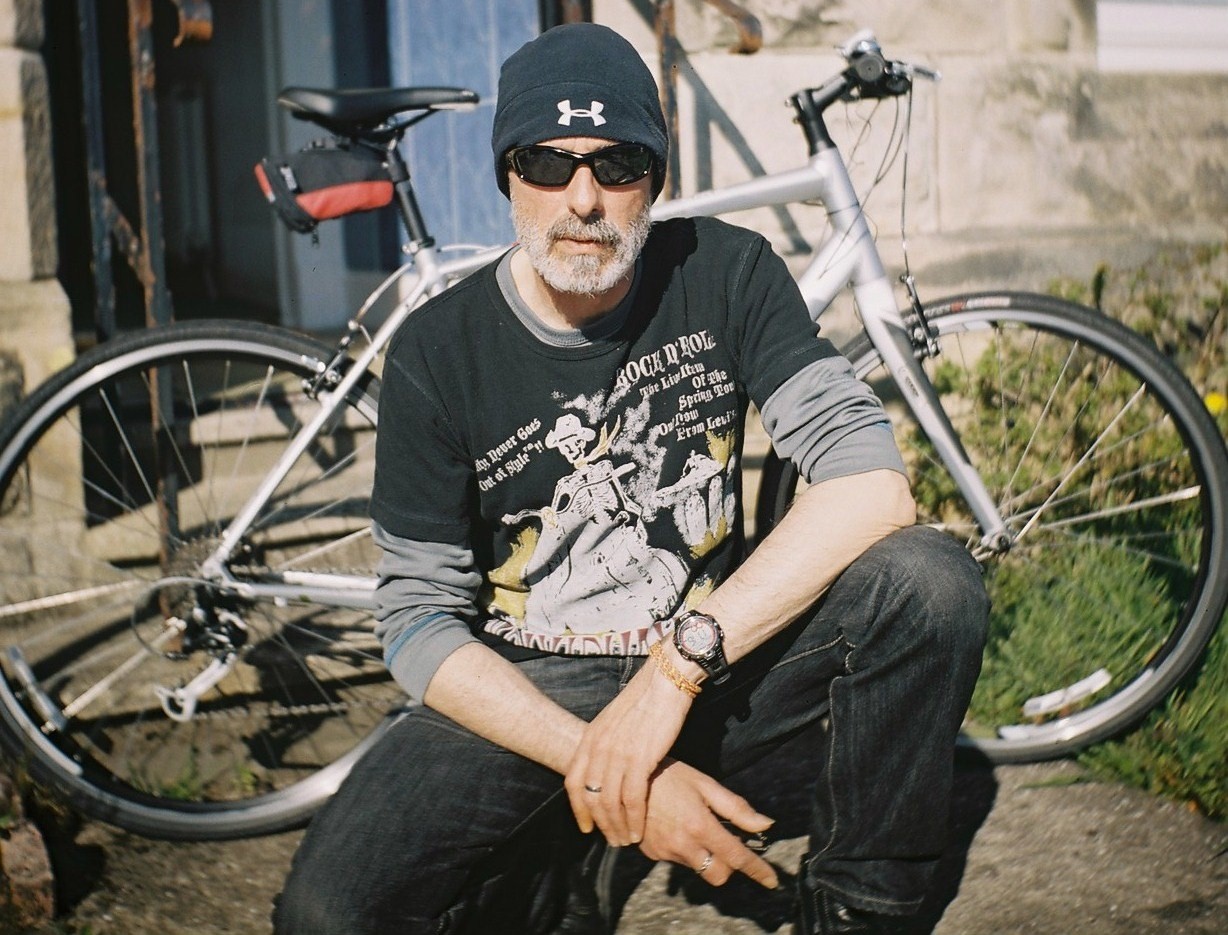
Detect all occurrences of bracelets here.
[647,643,703,700]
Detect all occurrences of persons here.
[275,23,994,935]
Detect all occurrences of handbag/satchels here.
[253,146,394,250]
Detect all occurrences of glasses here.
[502,142,655,187]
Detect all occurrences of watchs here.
[672,610,731,686]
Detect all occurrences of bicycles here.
[0,29,1228,841]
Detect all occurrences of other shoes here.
[794,854,908,935]
[546,845,620,934]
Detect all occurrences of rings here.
[696,857,714,876]
[586,784,601,794]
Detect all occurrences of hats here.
[490,22,669,205]
